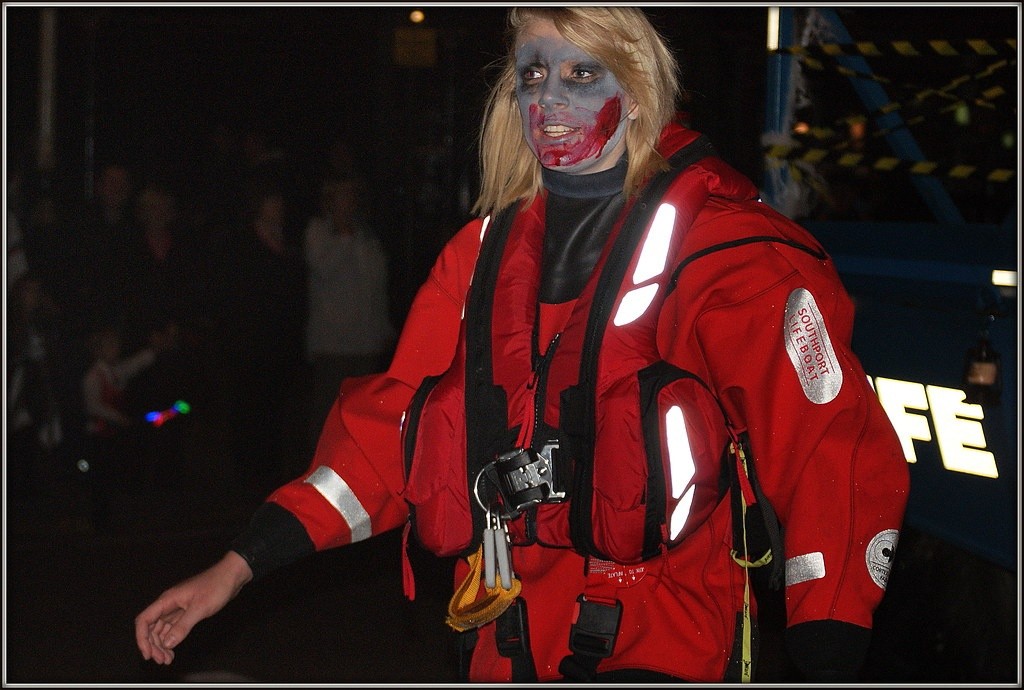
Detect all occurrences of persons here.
[136,5,910,683]
[78,164,401,531]
